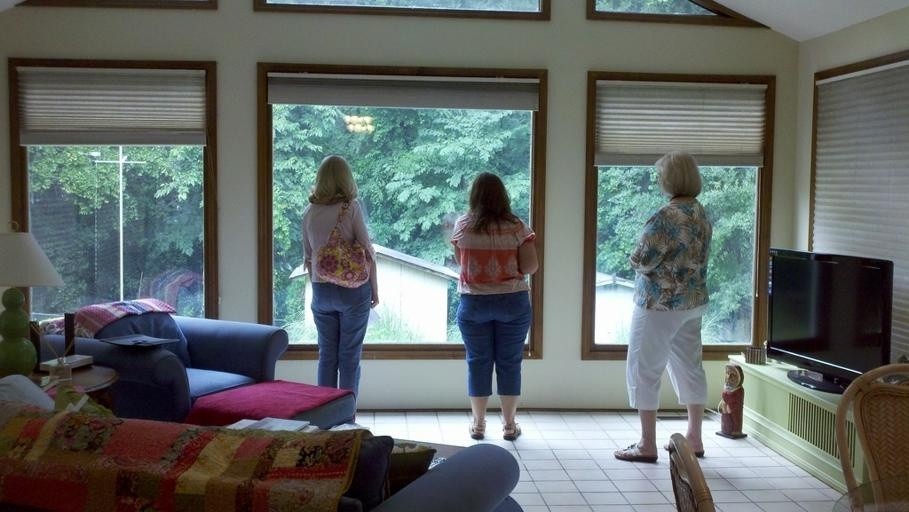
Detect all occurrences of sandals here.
[470,421,484,439]
[503,422,521,440]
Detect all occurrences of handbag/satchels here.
[316,201,373,288]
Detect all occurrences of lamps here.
[0,234,71,377]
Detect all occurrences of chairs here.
[833,362,904,512]
[665,432,724,512]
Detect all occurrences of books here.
[226,417,319,435]
[99,332,180,345]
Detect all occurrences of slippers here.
[665,443,704,457]
[614,444,658,463]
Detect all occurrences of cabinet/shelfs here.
[724,345,872,502]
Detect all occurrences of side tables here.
[32,363,120,431]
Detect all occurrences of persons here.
[615,152,711,460]
[302,155,379,391]
[450,174,538,441]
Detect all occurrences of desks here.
[831,474,909,512]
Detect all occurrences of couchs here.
[37,298,359,429]
[0,372,526,512]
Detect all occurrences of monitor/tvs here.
[763,248,893,394]
